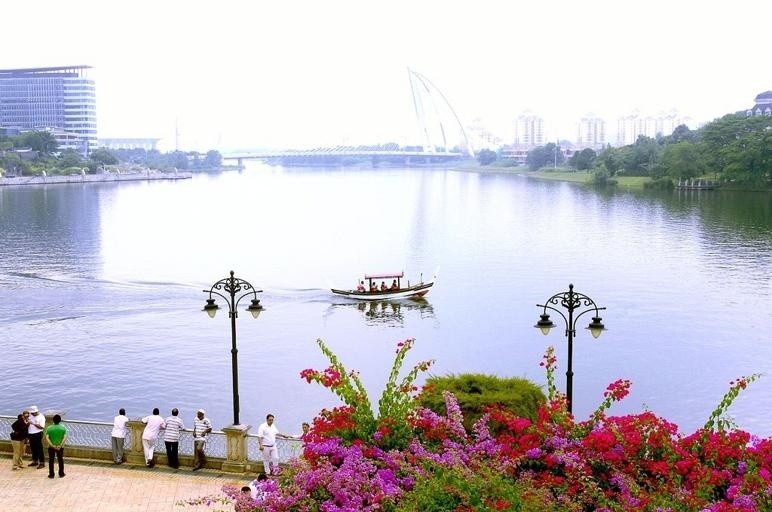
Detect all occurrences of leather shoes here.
[28,462,38,466]
[37,464,45,469]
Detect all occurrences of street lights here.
[201,271,266,425]
[533,284,609,414]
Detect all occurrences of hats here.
[28,406,39,413]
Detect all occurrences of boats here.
[331,271,434,300]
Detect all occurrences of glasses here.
[31,413,34,414]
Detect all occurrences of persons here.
[235,486,257,512]
[164,407,186,469]
[28,405,46,469]
[380,281,387,291]
[17,414,31,457]
[192,408,213,471]
[9,411,32,470]
[111,408,129,464]
[258,413,290,476]
[289,421,315,455]
[389,279,397,290]
[370,282,378,292]
[357,280,366,291]
[254,474,279,504]
[44,414,68,478]
[358,300,400,319]
[141,408,166,468]
